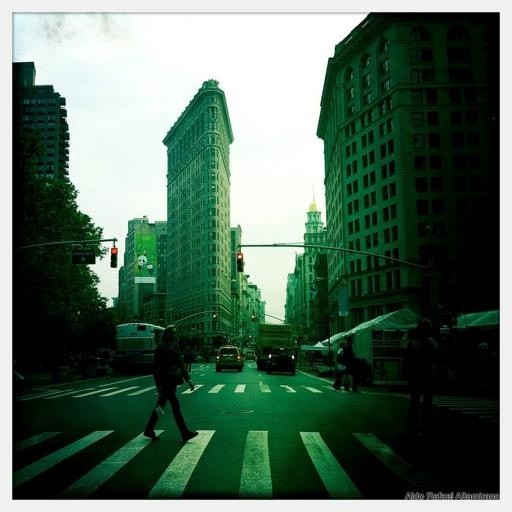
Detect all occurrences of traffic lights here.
[236,252,243,271]
[214,313,216,323]
[251,314,255,321]
[111,247,118,268]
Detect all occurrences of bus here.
[114,322,166,373]
[255,322,294,370]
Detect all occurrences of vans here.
[215,344,256,372]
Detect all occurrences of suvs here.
[267,346,296,376]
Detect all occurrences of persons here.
[332,336,359,392]
[403,318,439,405]
[184,347,192,372]
[144,325,198,442]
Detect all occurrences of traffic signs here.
[71,249,95,264]
